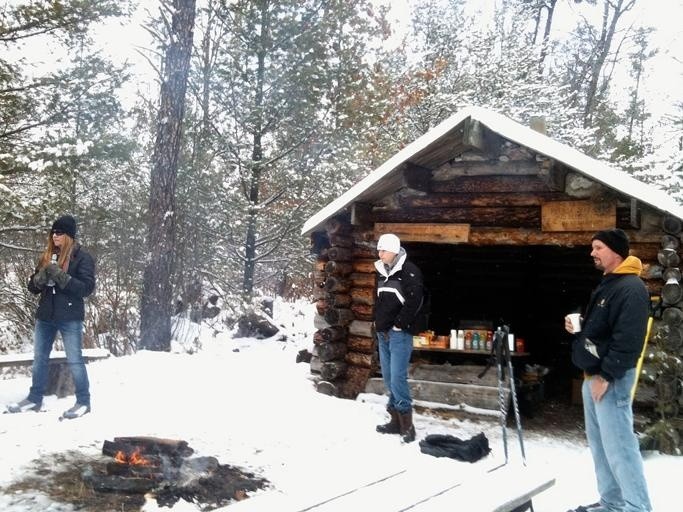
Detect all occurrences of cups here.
[568,312,581,333]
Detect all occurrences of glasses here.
[51,229,66,236]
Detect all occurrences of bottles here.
[46,254,57,287]
[450,326,506,353]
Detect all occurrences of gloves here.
[32,263,48,290]
[44,259,72,291]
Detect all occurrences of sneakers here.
[62,402,90,419]
[7,398,42,413]
[568,502,608,512]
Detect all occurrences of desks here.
[413,350,531,419]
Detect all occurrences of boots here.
[375,407,398,433]
[398,408,416,444]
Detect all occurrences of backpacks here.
[401,262,433,337]
[419,432,492,463]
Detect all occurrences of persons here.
[7,214,96,420]
[372,232,429,442]
[563,228,653,511]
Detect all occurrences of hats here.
[376,233,400,254]
[52,216,77,240]
[591,229,629,259]
